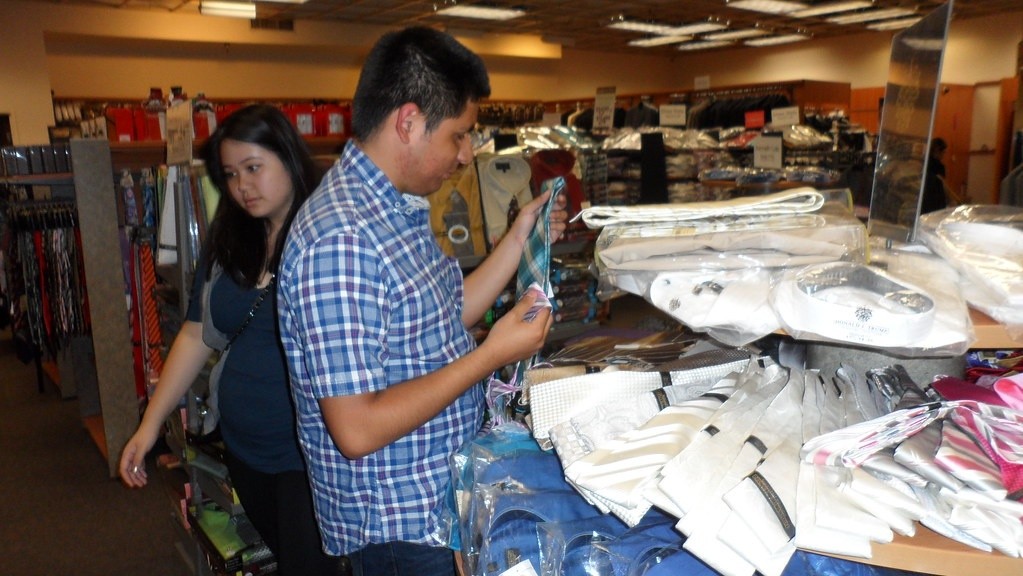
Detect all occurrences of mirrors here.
[867,0,953,244]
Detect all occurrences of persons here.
[118,103,323,576]
[275,23,571,576]
[920,137,948,214]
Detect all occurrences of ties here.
[571,187,827,240]
[489,280,552,402]
[513,177,567,386]
[528,349,1023,576]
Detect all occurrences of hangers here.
[15,201,78,231]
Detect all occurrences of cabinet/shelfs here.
[0,171,73,362]
[71,79,974,576]
